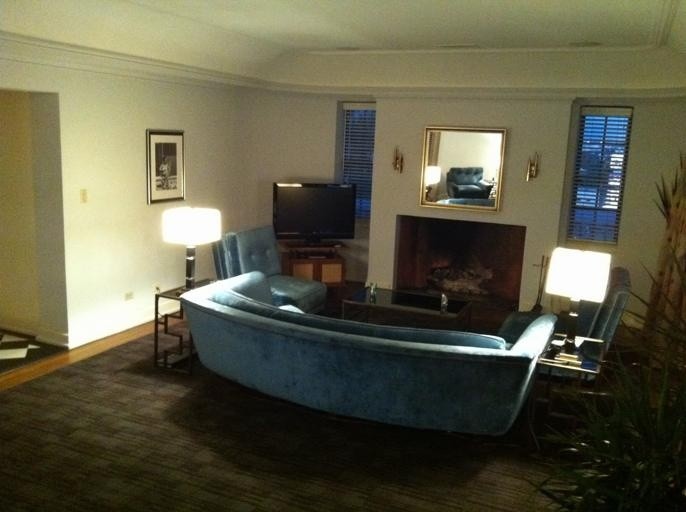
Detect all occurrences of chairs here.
[497,267,632,386]
[210,223,329,315]
[446,167,494,199]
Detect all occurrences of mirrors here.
[418,126,507,216]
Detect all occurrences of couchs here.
[177,272,560,452]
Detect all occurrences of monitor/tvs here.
[270,180,357,248]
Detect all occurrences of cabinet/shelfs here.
[279,240,350,289]
[152,278,217,376]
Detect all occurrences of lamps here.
[160,206,223,295]
[543,246,614,355]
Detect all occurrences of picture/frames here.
[146,128,186,205]
